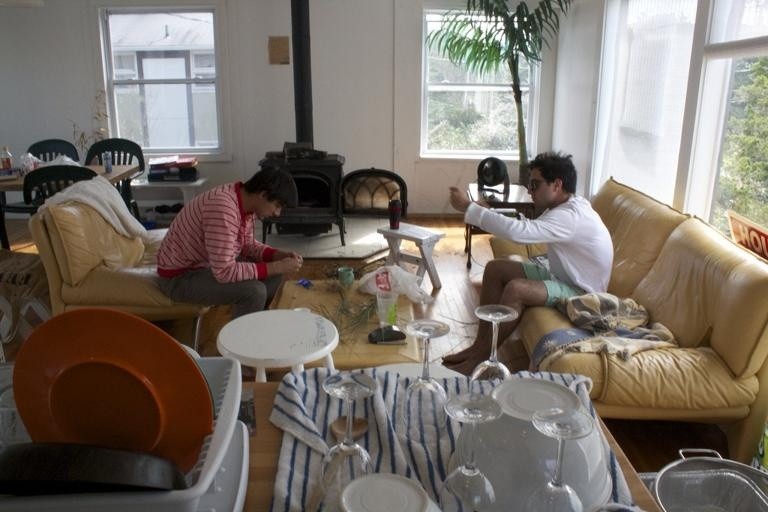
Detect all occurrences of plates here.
[8,304,219,482]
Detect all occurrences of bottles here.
[0,146,13,170]
[103,151,114,173]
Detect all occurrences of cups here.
[294,307,312,314]
[338,267,355,286]
[387,199,402,230]
[377,289,399,328]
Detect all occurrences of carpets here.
[256,217,389,259]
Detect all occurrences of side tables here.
[465,184,535,269]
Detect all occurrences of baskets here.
[1,351,245,512]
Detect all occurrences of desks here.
[241,382,664,512]
[115,179,207,206]
[1,165,140,250]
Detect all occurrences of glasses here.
[525,177,554,192]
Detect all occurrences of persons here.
[442,153,614,376]
[157,166,303,318]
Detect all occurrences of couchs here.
[490,177,768,465]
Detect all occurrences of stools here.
[377,222,445,289]
[217,309,339,382]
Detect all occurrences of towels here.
[270,367,647,512]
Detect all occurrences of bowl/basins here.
[336,473,444,512]
[0,438,190,496]
[451,377,614,512]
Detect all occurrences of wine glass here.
[441,394,504,512]
[522,409,594,512]
[320,374,382,511]
[393,320,451,452]
[467,303,522,402]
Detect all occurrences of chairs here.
[28,200,211,352]
[23,138,145,221]
